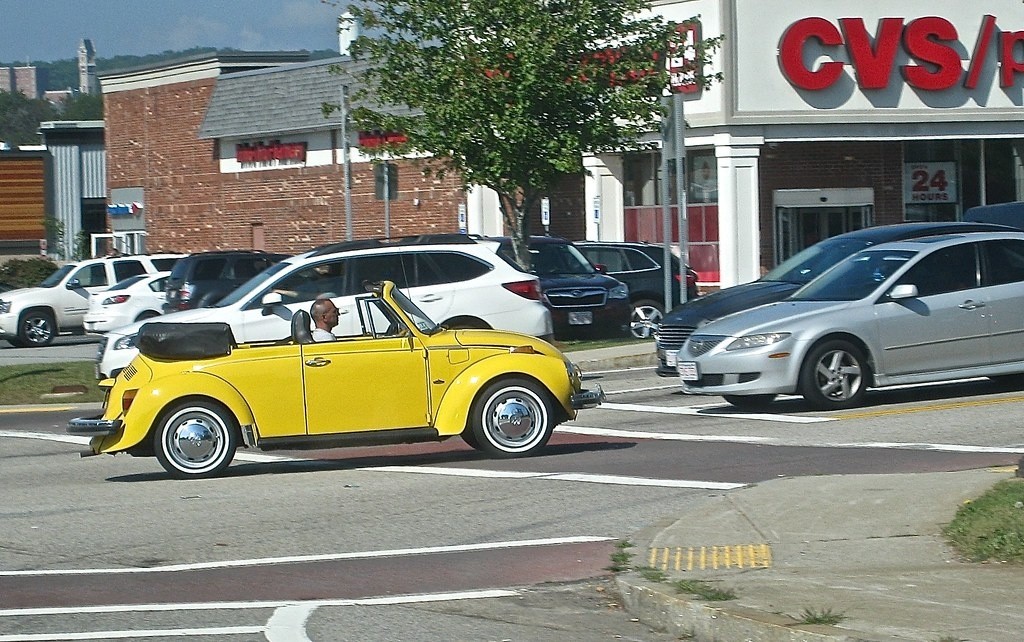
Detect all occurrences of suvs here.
[0,253,190,348]
[161,250,293,318]
[94,232,556,389]
[570,239,701,340]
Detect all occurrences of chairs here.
[292,309,314,344]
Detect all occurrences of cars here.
[653,201,1024,379]
[676,231,1024,411]
[488,233,631,337]
[80,271,176,337]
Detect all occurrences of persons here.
[310,299,340,342]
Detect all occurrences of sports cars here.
[63,280,607,481]
